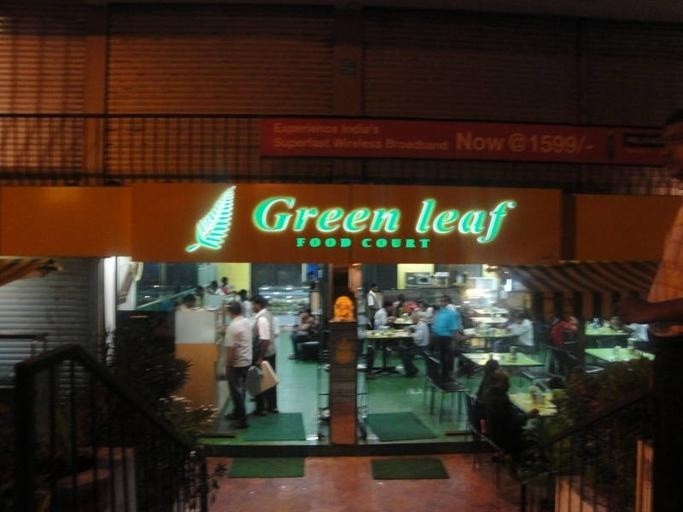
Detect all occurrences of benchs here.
[297,341,321,360]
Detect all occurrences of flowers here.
[158,393,220,440]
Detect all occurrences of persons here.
[160,275,278,429]
[366,282,648,465]
[288,307,321,359]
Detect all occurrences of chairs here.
[423,349,468,425]
[464,391,492,469]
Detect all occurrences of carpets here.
[371,458,449,480]
[243,413,309,441]
[364,412,436,441]
[227,457,306,478]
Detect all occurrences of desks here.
[461,305,656,418]
[357,314,416,379]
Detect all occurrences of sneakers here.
[288,353,297,360]
[225,407,279,429]
[402,369,419,378]
